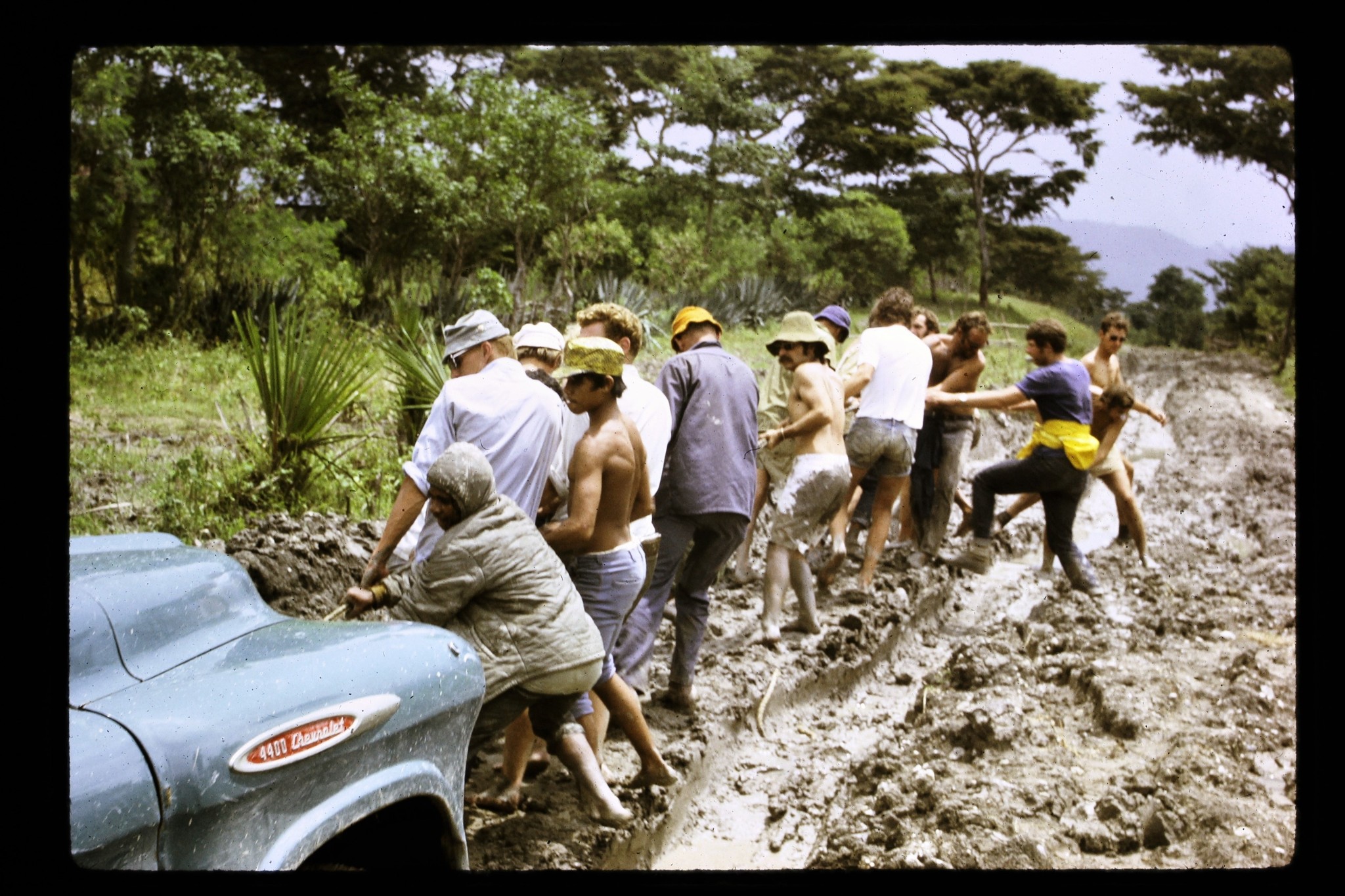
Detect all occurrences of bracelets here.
[780,428,787,440]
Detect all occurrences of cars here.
[68,529,487,870]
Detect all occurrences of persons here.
[922,320,1101,599]
[479,338,678,806]
[1000,309,1166,573]
[814,287,991,596]
[504,302,761,772]
[342,440,634,822]
[733,304,851,584]
[359,307,566,587]
[742,310,850,648]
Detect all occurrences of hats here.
[551,337,626,379]
[766,312,830,358]
[814,303,851,344]
[512,321,565,352]
[441,309,510,366]
[670,306,723,353]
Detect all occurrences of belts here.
[641,538,661,553]
[924,411,975,421]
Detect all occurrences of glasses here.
[446,350,467,371]
[776,341,801,351]
[1107,330,1126,343]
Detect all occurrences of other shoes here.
[937,536,989,575]
[1070,574,1105,597]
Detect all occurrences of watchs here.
[959,392,968,406]
